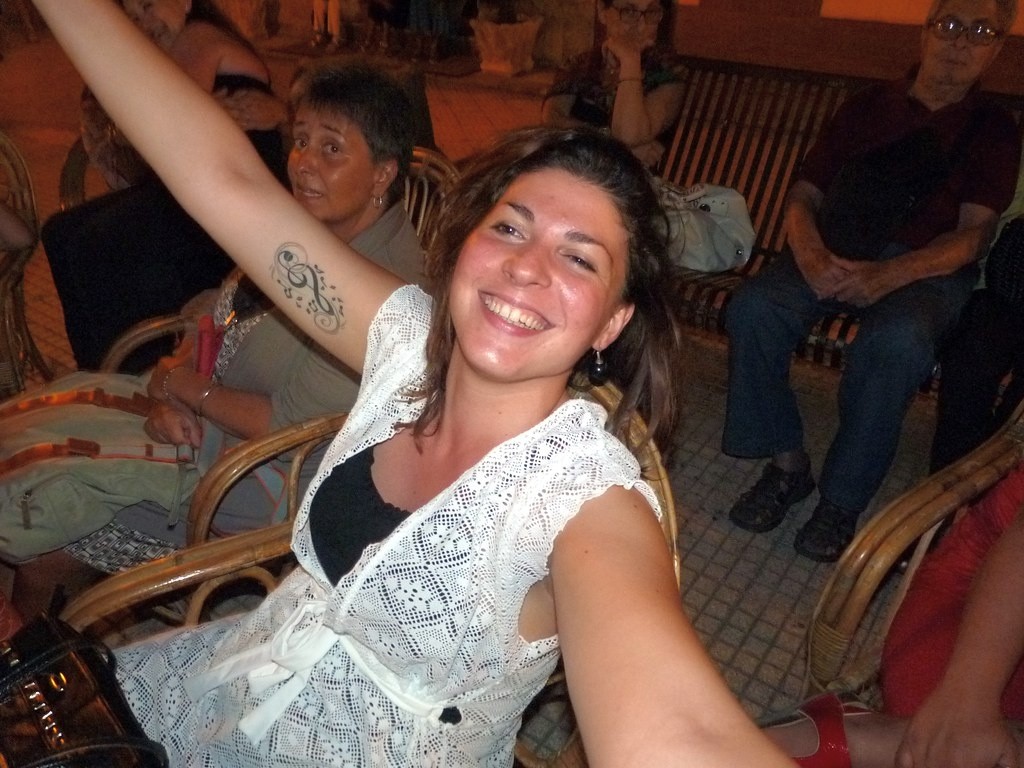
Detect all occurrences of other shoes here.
[793,495,859,565]
[358,38,392,58]
[305,32,350,56]
[409,44,443,65]
[727,452,817,533]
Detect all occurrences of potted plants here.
[470,0,543,76]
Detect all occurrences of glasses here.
[608,3,665,25]
[928,15,1004,46]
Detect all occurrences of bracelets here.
[162,366,182,402]
[617,77,643,83]
[195,381,223,416]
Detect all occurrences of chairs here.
[59,356,684,768]
[101,147,465,630]
[0,120,91,403]
[801,399,1024,711]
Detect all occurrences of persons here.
[723,0,1024,575]
[540,0,689,170]
[756,467,1024,768]
[0,0,441,629]
[0,0,799,768]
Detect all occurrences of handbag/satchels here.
[0,370,201,569]
[650,178,758,277]
[0,611,169,768]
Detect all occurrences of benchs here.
[661,55,1024,423]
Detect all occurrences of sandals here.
[758,688,875,768]
